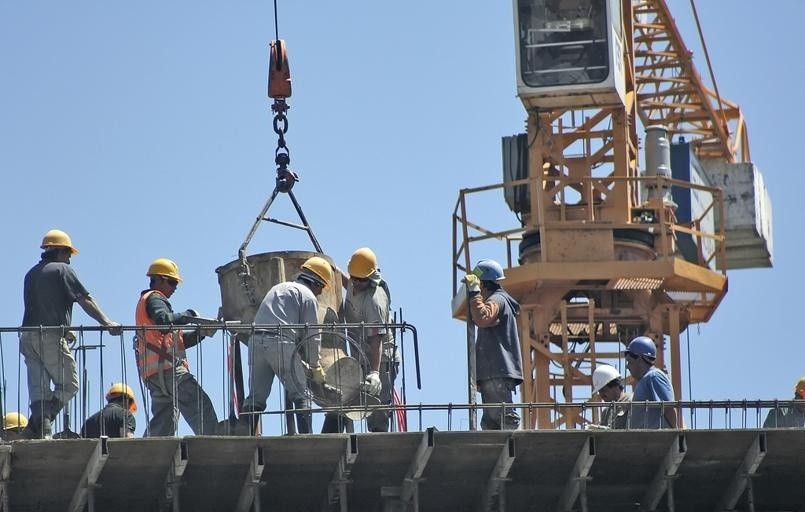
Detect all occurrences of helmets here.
[106,382,137,415]
[40,229,78,256]
[592,364,622,396]
[300,256,333,290]
[347,246,378,278]
[622,336,657,359]
[146,257,183,282]
[797,379,805,400]
[2,411,30,429]
[473,259,506,281]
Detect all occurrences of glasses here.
[162,275,179,286]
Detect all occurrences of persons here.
[19,229,123,439]
[321,247,400,433]
[80,382,138,438]
[462,259,524,430]
[582,364,634,429]
[620,335,680,428]
[233,257,334,436]
[763,375,805,428]
[135,259,220,436]
[0,411,29,441]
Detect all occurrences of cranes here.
[258,1,776,433]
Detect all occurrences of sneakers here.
[20,426,52,440]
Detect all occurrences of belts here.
[254,328,277,336]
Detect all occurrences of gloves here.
[314,367,327,384]
[366,370,382,396]
[464,273,482,292]
